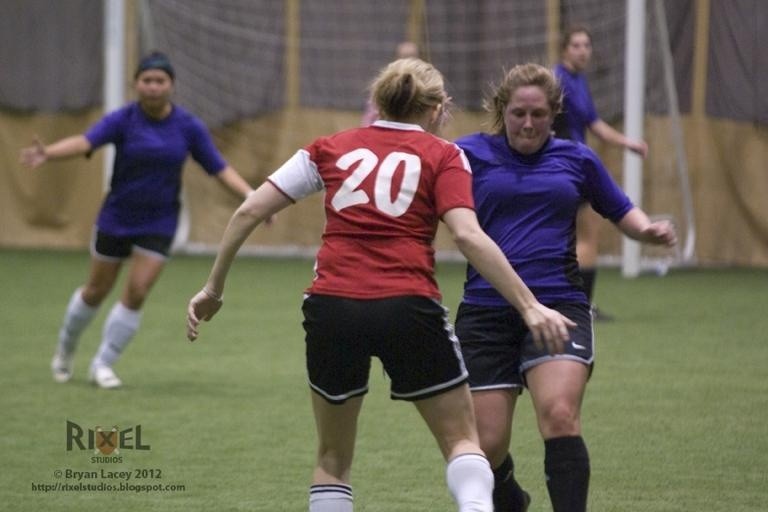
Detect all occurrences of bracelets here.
[201,287,224,302]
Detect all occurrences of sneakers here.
[588,303,618,325]
[52,345,75,384]
[86,360,123,391]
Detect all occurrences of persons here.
[359,38,421,128]
[449,59,680,512]
[189,57,580,512]
[546,25,652,322]
[16,47,280,390]
